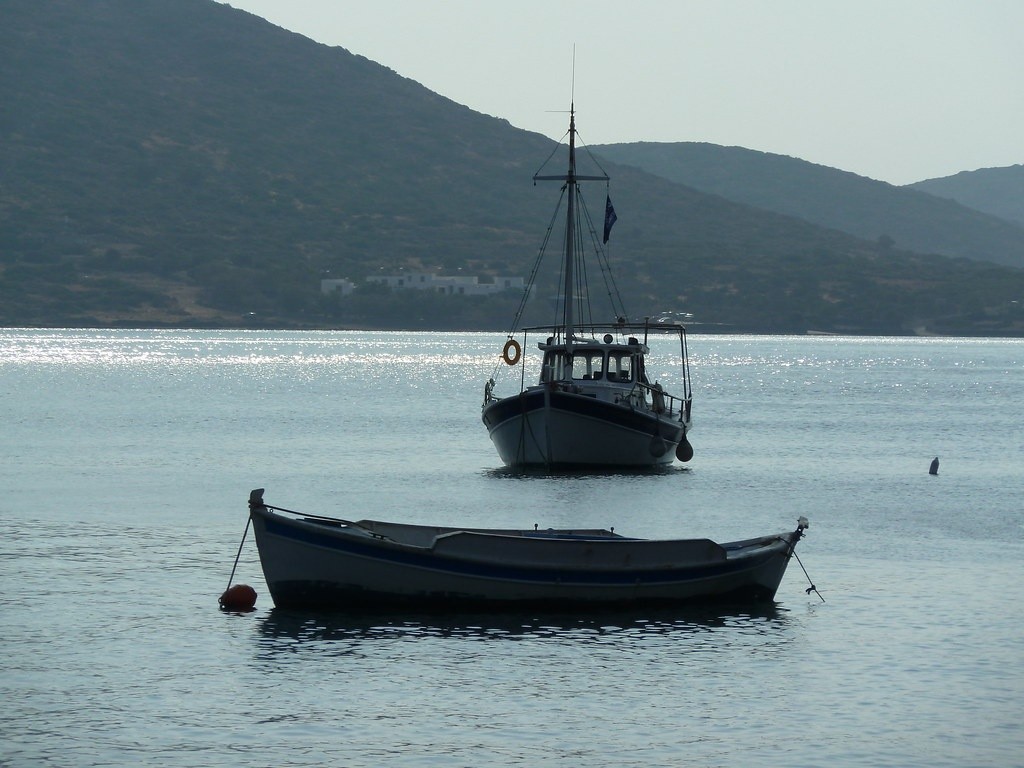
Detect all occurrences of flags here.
[603,196,617,244]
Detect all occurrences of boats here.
[247,488,809,618]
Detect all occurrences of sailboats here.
[481,41,693,472]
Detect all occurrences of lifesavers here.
[502,339,523,366]
